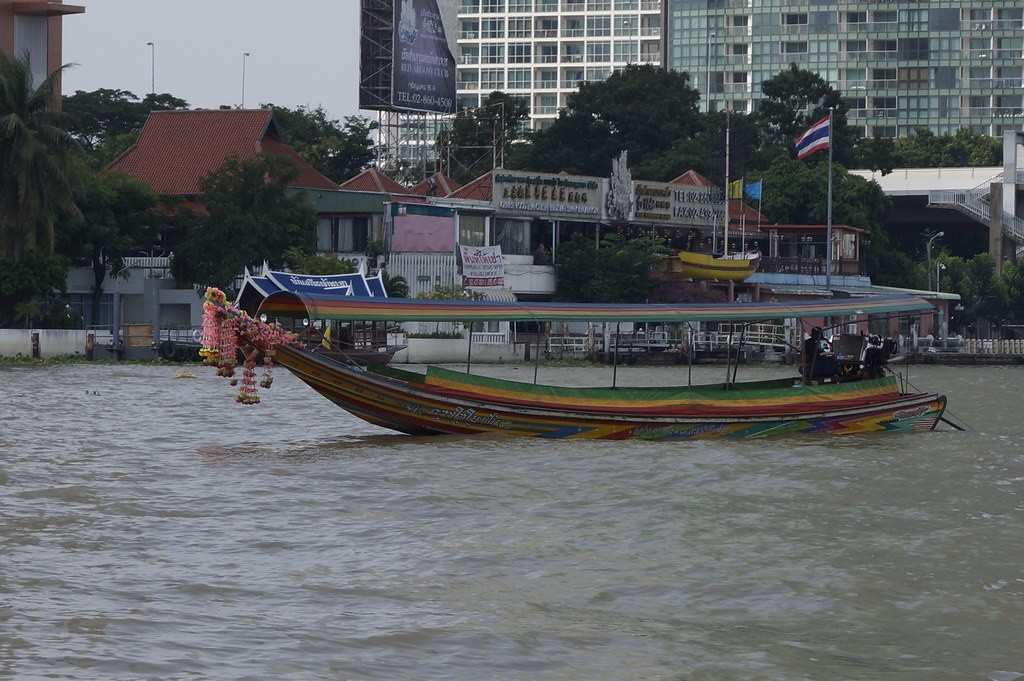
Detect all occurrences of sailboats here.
[678,129,762,281]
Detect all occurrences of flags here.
[729,179,743,198]
[744,182,761,200]
[794,115,830,159]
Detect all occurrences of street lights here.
[937,262,946,293]
[929,232,944,290]
[548,220,555,266]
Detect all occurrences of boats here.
[902,351,937,364]
[582,349,639,364]
[150,262,408,367]
[641,348,748,366]
[206,286,945,437]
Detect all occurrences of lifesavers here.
[161,340,176,359]
[192,329,202,344]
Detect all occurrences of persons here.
[799,326,832,377]
